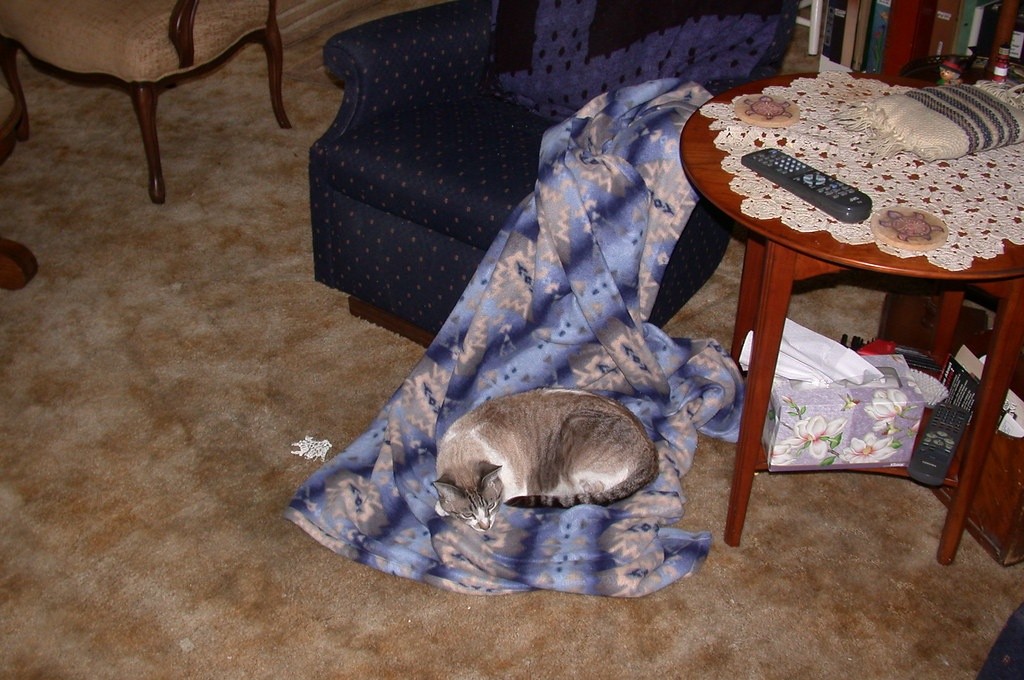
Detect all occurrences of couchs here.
[308,0,801,353]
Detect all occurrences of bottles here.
[998,41,1010,56]
[1009,15,1024,64]
[992,54,1010,84]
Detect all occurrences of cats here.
[431,387,658,533]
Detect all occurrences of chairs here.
[0,0,293,206]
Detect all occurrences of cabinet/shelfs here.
[875,0,1024,565]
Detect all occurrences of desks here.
[680,72,1024,566]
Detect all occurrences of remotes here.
[906,403,970,486]
[740,147,872,224]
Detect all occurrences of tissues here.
[739,317,925,472]
